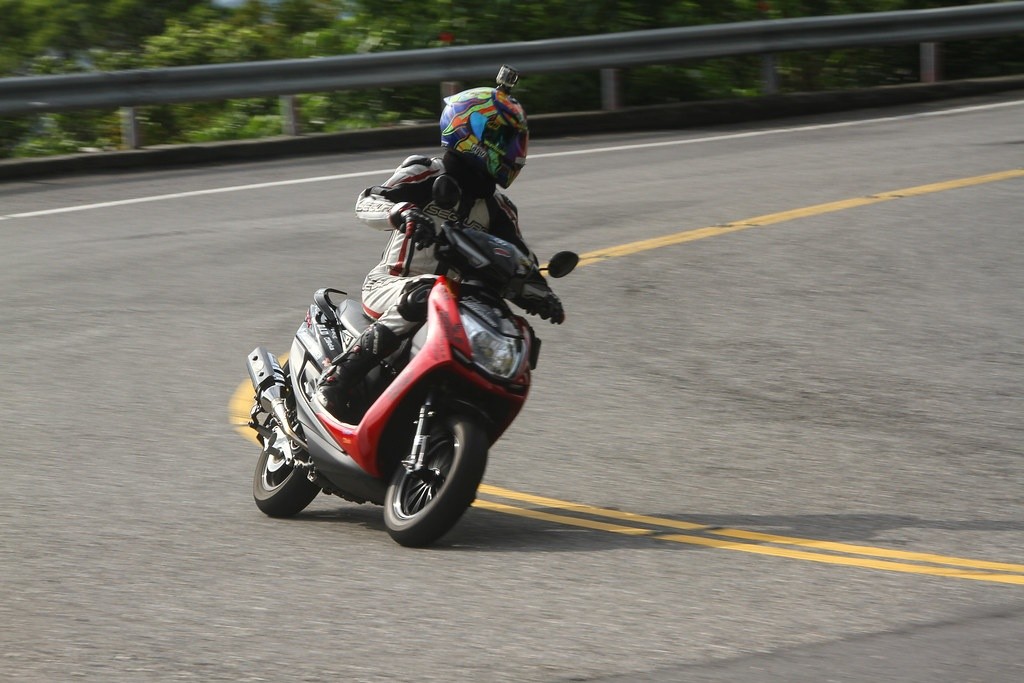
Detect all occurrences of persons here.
[314,86,565,410]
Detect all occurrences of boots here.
[315,323,401,413]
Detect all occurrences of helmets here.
[439,88,529,190]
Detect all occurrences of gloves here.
[527,281,564,324]
[389,201,436,251]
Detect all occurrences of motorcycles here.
[245,173,579,547]
